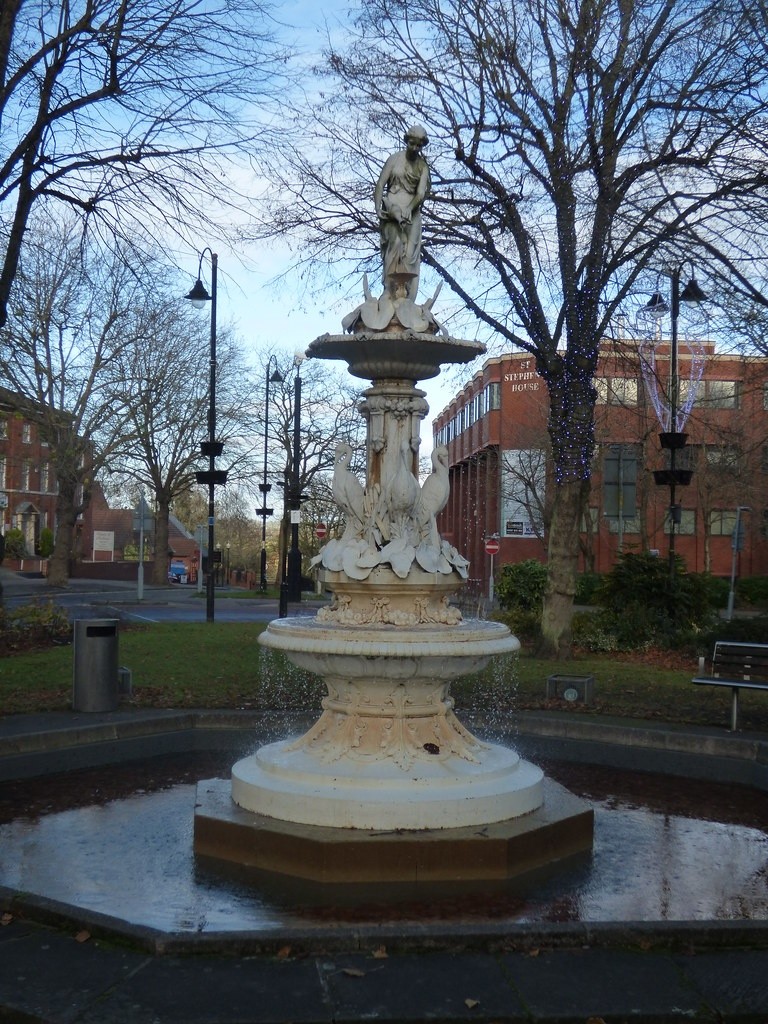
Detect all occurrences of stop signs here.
[316,524,326,537]
[486,540,500,555]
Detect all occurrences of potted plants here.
[40,528,55,576]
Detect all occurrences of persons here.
[374,125,430,302]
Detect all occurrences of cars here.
[170,561,188,582]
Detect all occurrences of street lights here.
[259,348,284,596]
[286,351,314,600]
[187,247,221,624]
[216,541,222,552]
[226,542,231,586]
[724,504,754,625]
[641,251,708,579]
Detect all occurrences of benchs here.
[691,642,768,733]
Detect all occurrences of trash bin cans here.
[71,616,121,713]
